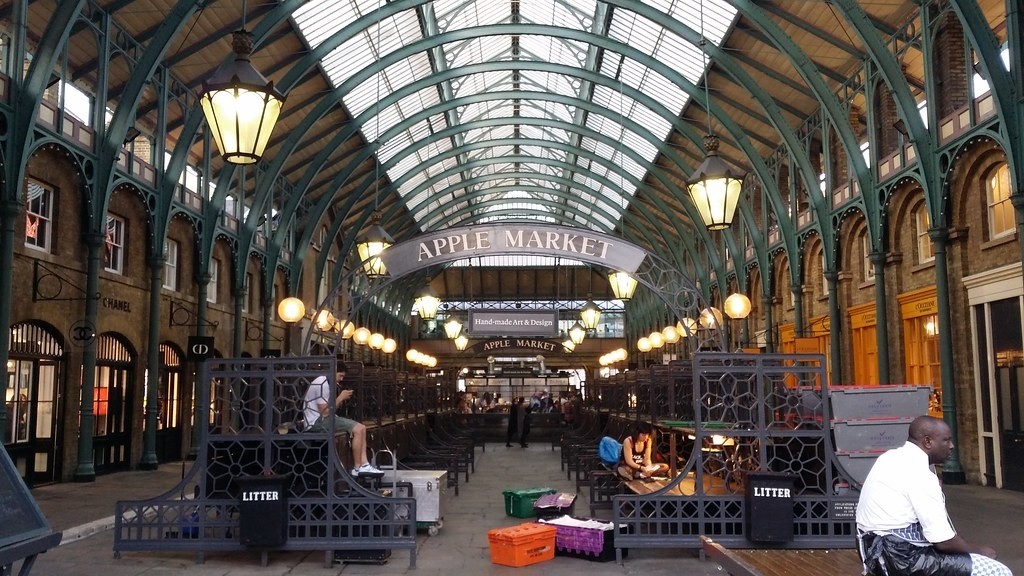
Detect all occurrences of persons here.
[855,415,1012,576]
[303,361,384,479]
[465,391,576,448]
[614,421,670,481]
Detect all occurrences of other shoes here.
[506,442,514,447]
[521,442,529,448]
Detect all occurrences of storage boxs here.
[536,515,634,563]
[502,487,557,519]
[487,522,557,567]
[375,470,448,521]
[532,493,578,520]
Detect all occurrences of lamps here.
[563,336,576,354]
[353,0,395,279]
[196,0,286,166]
[892,119,910,138]
[597,347,629,375]
[442,262,463,339]
[685,0,748,231]
[452,335,469,352]
[607,54,639,301]
[637,291,752,353]
[413,123,442,322]
[406,349,438,368]
[310,309,397,354]
[567,261,588,345]
[124,127,140,150]
[278,297,307,324]
[580,262,603,336]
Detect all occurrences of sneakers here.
[351,468,360,477]
[359,463,384,477]
